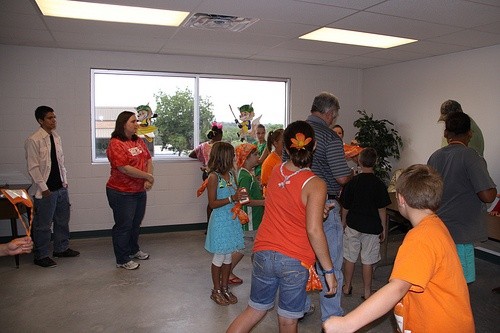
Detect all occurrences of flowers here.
[289,132,313,150]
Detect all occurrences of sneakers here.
[117,261,140,270]
[130,252,150,260]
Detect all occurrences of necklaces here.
[448,141,466,146]
[278,161,311,188]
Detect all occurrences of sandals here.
[211,288,238,306]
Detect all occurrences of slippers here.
[228,276,243,284]
[342,285,353,298]
[362,289,377,301]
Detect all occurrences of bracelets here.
[228,196,233,204]
[231,195,235,202]
[323,269,335,275]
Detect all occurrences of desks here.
[379,192,399,266]
[0,184,32,266]
[474,213,500,257]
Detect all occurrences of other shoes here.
[35,257,55,267]
[53,248,80,257]
[299,304,314,322]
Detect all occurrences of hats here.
[437,99,462,122]
[235,143,257,168]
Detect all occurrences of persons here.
[106,112,155,270]
[322,165,475,333]
[25,106,79,267]
[188,93,393,333]
[427,112,497,283]
[0,236,34,257]
[438,99,484,155]
